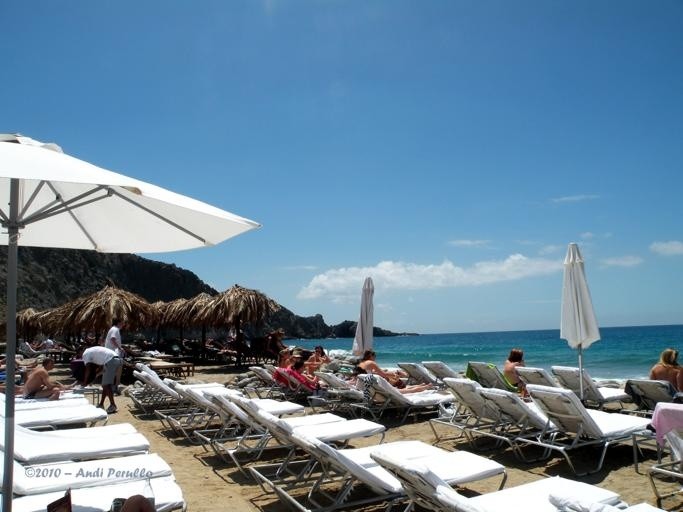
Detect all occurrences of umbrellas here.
[10,281,286,363]
[351,276,377,355]
[0,132,263,512]
[559,242,600,402]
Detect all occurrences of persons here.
[224,344,436,396]
[504,346,526,388]
[105,317,126,396]
[75,343,120,414]
[648,348,683,397]
[113,495,153,512]
[0,321,73,401]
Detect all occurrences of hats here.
[293,351,303,358]
[75,344,84,360]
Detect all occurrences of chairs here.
[0,339,683,512]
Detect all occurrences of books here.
[47,486,73,512]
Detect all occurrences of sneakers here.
[97,405,118,414]
